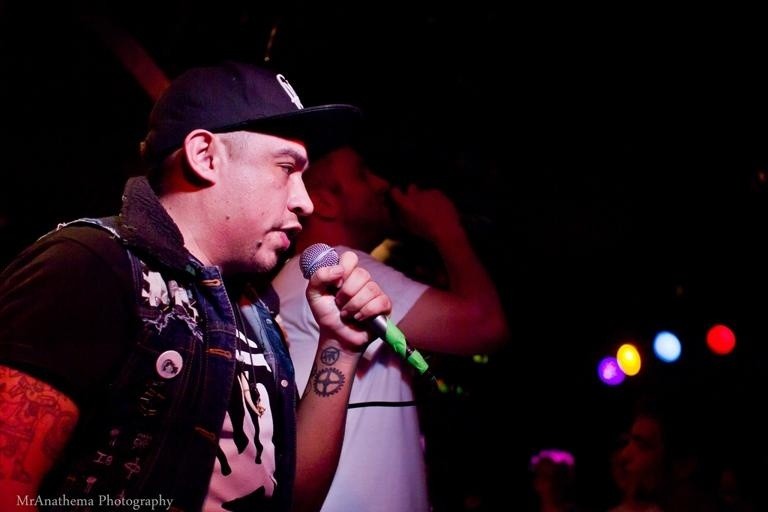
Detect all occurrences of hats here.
[138,60,367,168]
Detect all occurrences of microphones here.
[298,242,435,380]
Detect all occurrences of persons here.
[267,140,513,512]
[1,58,398,510]
[531,390,740,512]
